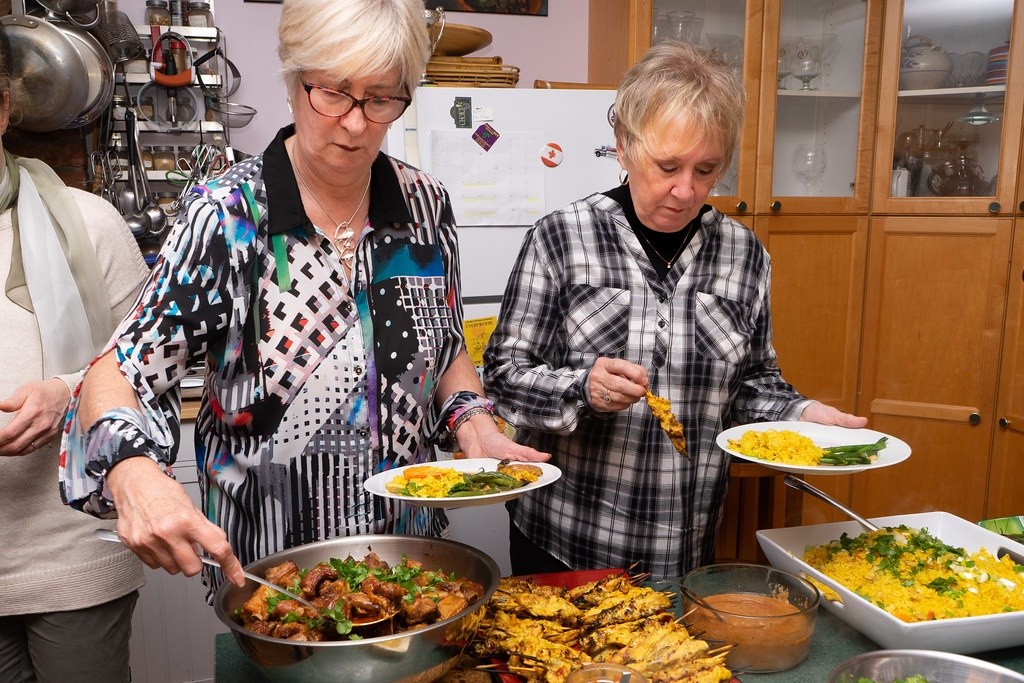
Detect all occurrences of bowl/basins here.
[680,563,821,675]
[944,49,989,88]
[213,534,501,682]
[824,649,1024,683]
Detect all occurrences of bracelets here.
[451,409,498,442]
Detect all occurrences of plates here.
[716,422,912,475]
[363,459,563,507]
[755,511,1024,654]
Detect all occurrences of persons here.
[482,40,870,583]
[0,19,151,683]
[56,1,554,605]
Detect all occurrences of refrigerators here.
[388,86,628,385]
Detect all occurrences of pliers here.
[91,149,124,215]
[177,145,210,182]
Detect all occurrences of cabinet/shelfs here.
[586,1,1024,564]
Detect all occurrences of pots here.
[0,1,116,131]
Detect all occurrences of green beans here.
[818,436,888,465]
[448,467,527,497]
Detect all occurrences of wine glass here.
[778,35,822,90]
[795,144,826,197]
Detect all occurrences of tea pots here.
[928,167,997,198]
[899,34,952,92]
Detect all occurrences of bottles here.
[144,0,171,26]
[169,0,189,27]
[188,2,213,27]
[132,96,154,121]
[166,96,195,123]
[157,192,179,227]
[111,95,130,120]
[109,144,222,170]
[170,42,186,75]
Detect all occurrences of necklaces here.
[640,225,693,268]
[292,143,371,271]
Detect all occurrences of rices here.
[387,466,465,498]
[791,533,1024,623]
[728,427,831,467]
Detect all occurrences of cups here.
[650,7,743,77]
[897,122,978,165]
[891,170,912,196]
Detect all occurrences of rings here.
[30,442,37,450]
[604,390,611,403]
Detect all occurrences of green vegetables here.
[802,523,1024,615]
[399,482,418,495]
[237,555,458,639]
[858,674,928,683]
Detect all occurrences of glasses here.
[300,73,413,124]
